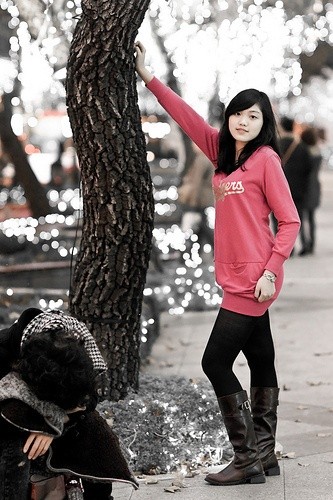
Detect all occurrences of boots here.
[204,389,266,487]
[248,386,282,477]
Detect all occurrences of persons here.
[134,41,301,488]
[177,103,320,257]
[0,309,138,500]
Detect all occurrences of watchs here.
[263,272,276,282]
